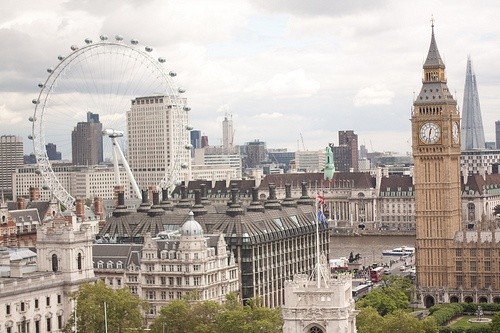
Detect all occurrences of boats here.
[382,245,415,256]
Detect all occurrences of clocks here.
[452,121,459,144]
[419,122,441,145]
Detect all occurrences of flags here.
[317,192,326,206]
[317,209,328,225]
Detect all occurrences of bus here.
[371,266,384,282]
[351,285,368,301]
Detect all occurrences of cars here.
[400,265,405,271]
[384,270,389,275]
[365,281,374,286]
